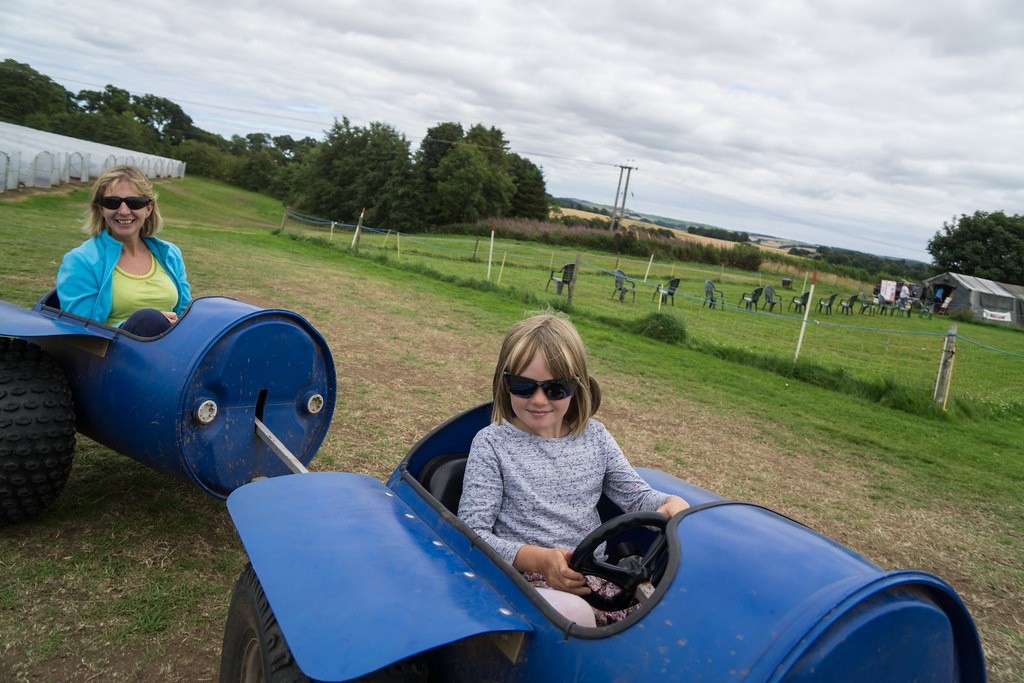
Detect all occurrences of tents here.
[923,272,1024,332]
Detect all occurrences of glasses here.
[99,196,150,210]
[503,370,580,399]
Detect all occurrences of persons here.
[455,314,689,627]
[54,165,192,338]
[900,281,944,321]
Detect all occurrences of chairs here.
[702,280,724,310]
[877,294,894,317]
[611,270,635,304]
[763,286,782,315]
[738,288,763,312]
[836,294,858,316]
[860,292,875,317]
[788,292,809,313]
[546,264,575,295]
[814,293,838,315]
[414,450,467,514]
[652,278,680,307]
